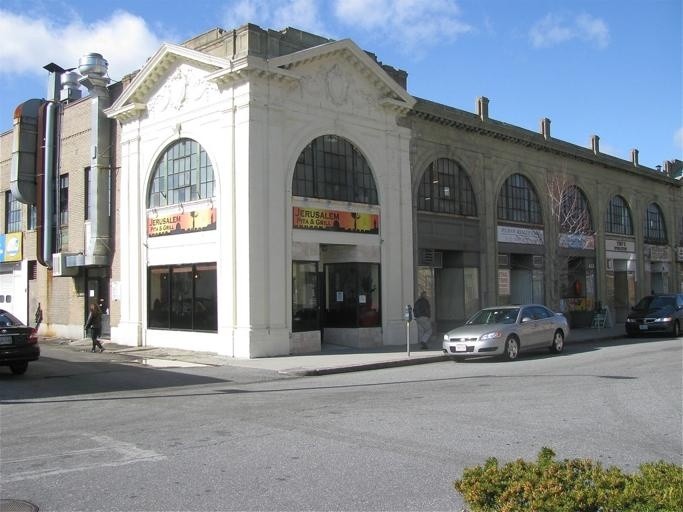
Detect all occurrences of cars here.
[0,309,41,377]
[437,303,569,362]
[148,298,213,328]
[621,292,682,337]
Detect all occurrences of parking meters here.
[404,303,413,355]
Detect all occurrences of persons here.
[84,302,105,353]
[413,291,434,350]
